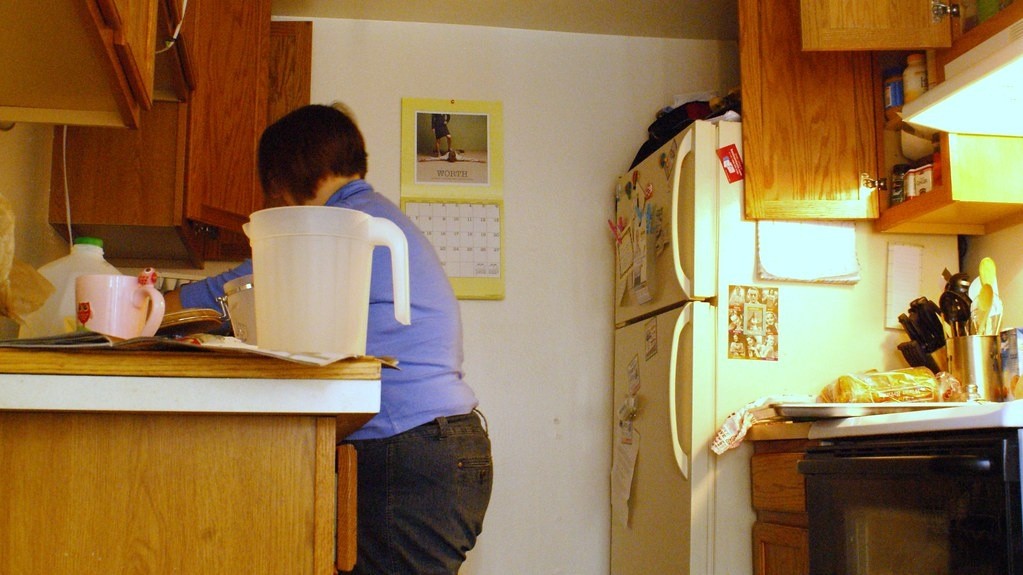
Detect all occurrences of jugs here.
[242,206,410,361]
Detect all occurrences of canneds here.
[883,66,904,120]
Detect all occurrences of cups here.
[223,274,256,347]
[945,335,1005,404]
[74,273,165,340]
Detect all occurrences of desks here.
[0,349,382,575]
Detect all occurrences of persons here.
[162,104,493,575]
[729,285,778,358]
[418,114,486,163]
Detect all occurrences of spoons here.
[940,257,1003,335]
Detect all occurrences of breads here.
[821,366,940,404]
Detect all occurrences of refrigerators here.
[610,121,962,575]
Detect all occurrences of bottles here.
[19,237,124,340]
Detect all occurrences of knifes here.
[897,297,945,376]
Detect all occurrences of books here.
[0,334,401,370]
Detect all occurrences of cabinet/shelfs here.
[746,421,821,575]
[737,0,1023,237]
[0,0,312,270]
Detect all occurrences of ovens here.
[797,437,1018,575]
